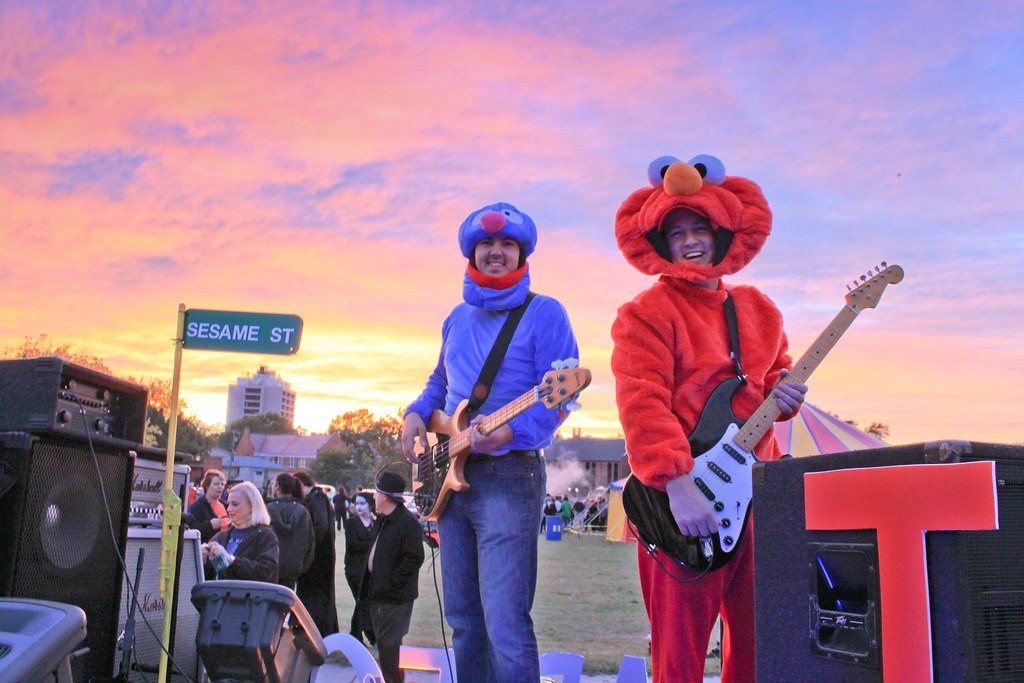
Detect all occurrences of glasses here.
[356,501,367,506]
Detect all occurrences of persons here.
[609,155,808,683]
[184,468,337,639]
[350,485,365,503]
[343,492,380,647]
[540,493,608,533]
[402,203,579,683]
[367,472,425,683]
[333,485,348,530]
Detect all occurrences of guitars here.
[412,358,594,521]
[623,260,904,573]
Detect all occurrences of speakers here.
[0,597,88,683]
[0,431,137,683]
[190,580,328,683]
[751,439,1024,683]
[112,527,207,683]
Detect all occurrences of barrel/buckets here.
[545,516,562,541]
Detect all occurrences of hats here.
[377,472,406,502]
